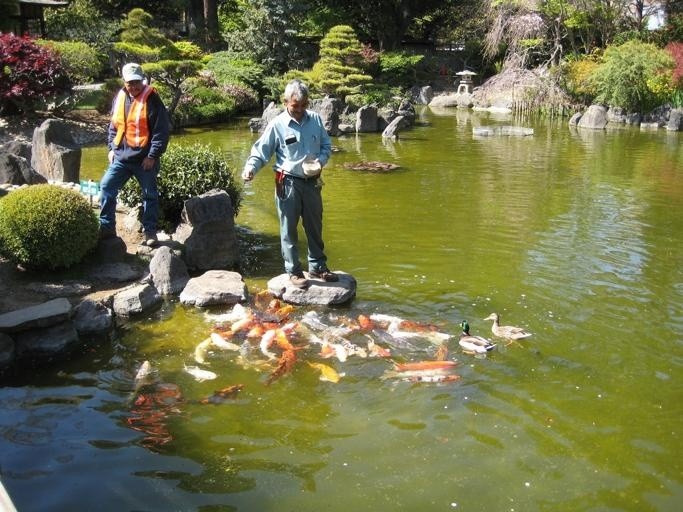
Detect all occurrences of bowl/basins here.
[301,159,321,175]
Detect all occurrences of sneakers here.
[288,272,308,288]
[308,269,338,282]
[143,223,157,246]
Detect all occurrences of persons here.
[98,63,169,247]
[241,78,338,289]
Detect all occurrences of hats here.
[121,62,143,82]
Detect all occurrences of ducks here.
[483,312,537,349]
[458,320,497,354]
